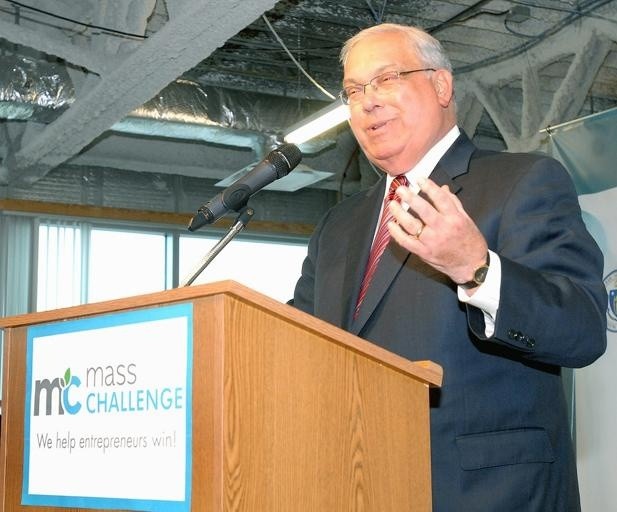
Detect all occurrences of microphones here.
[185,145,302,233]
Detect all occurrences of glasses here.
[339,67,436,106]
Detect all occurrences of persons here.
[276,23,608,510]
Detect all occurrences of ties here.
[352,173,409,320]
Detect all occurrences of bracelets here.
[456,251,489,292]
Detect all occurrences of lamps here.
[284,96,351,146]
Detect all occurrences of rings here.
[413,224,424,237]
